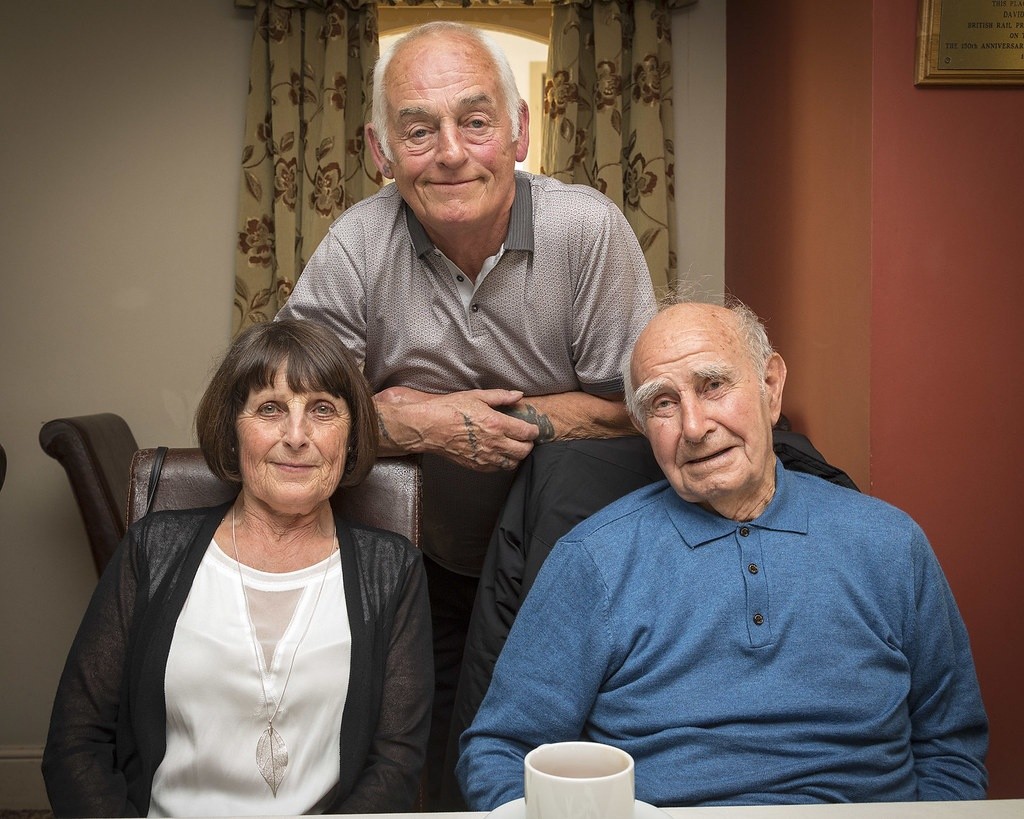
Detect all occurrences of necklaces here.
[231,504,338,799]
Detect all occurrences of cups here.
[484,797,675,819]
[524,741,634,819]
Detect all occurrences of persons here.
[275,22,661,475]
[40,321,436,819]
[454,302,993,811]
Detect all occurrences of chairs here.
[519,430,816,585]
[40,411,137,571]
[123,448,425,550]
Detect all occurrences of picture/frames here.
[913,0,1024,89]
